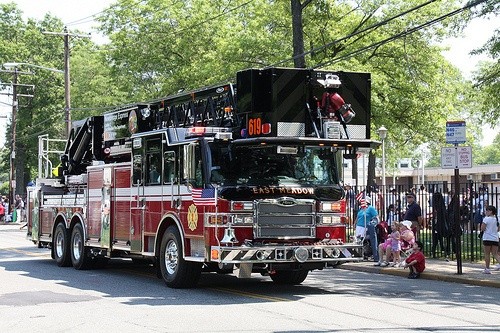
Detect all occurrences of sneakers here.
[483,268,490,274]
[408,272,412,278]
[411,272,417,279]
[494,265,500,270]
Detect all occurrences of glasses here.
[406,196,413,198]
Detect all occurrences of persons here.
[403,193,422,230]
[353,196,378,261]
[0,194,27,222]
[404,241,425,279]
[480,206,500,273]
[375,220,415,267]
[461,187,488,238]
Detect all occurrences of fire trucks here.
[24,68,382,288]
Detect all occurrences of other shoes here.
[380,261,388,266]
[374,261,380,266]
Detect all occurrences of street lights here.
[378,124,388,220]
[3,63,72,136]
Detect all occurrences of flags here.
[356,190,367,208]
[192,189,215,205]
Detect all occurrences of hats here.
[406,192,416,198]
[400,220,412,229]
[365,195,371,204]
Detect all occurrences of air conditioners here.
[491,172,500,180]
